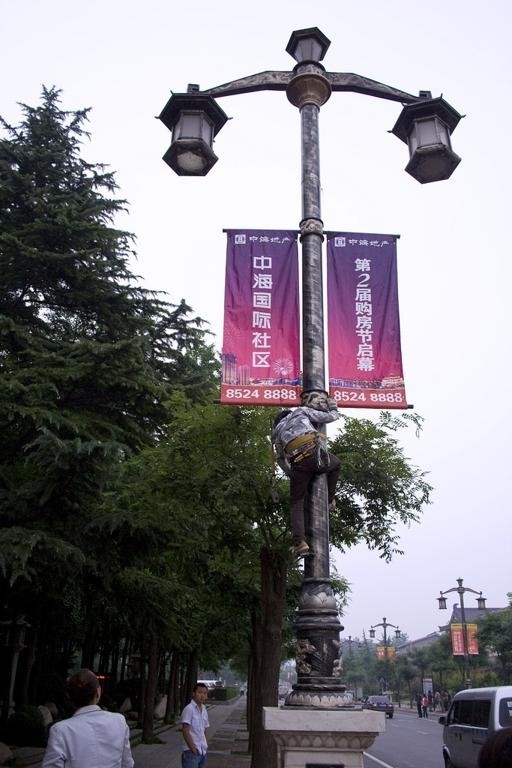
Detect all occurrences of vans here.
[196,679,225,691]
[438,684,512,768]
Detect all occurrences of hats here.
[63,670,105,702]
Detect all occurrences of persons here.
[40,668,136,768]
[415,687,452,720]
[179,682,210,767]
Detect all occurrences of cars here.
[278,682,294,701]
[361,694,396,719]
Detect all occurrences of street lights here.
[157,17,464,700]
[435,576,488,689]
[338,634,364,659]
[368,616,402,691]
